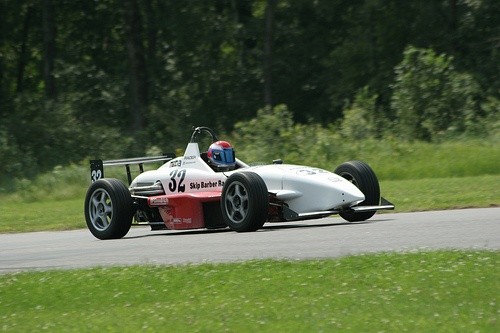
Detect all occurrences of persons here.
[208,141,236,172]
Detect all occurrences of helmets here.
[208,140,236,168]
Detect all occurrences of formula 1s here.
[83,126,394,240]
